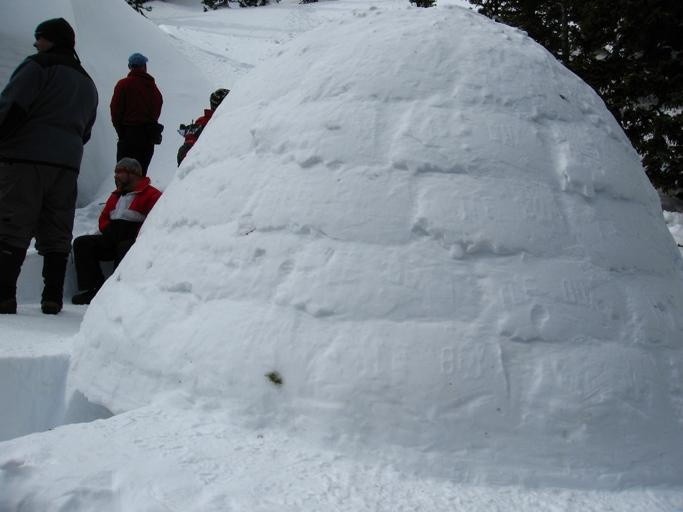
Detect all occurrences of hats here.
[35,18,76,47]
[210,89,231,111]
[115,157,142,177]
[128,54,147,67]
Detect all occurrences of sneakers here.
[1,299,16,314]
[41,301,61,314]
[72,290,98,304]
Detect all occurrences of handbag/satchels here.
[154,123,164,144]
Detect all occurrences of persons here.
[176,88,231,166]
[109,53,162,176]
[0,18,97,315]
[67,157,161,306]
